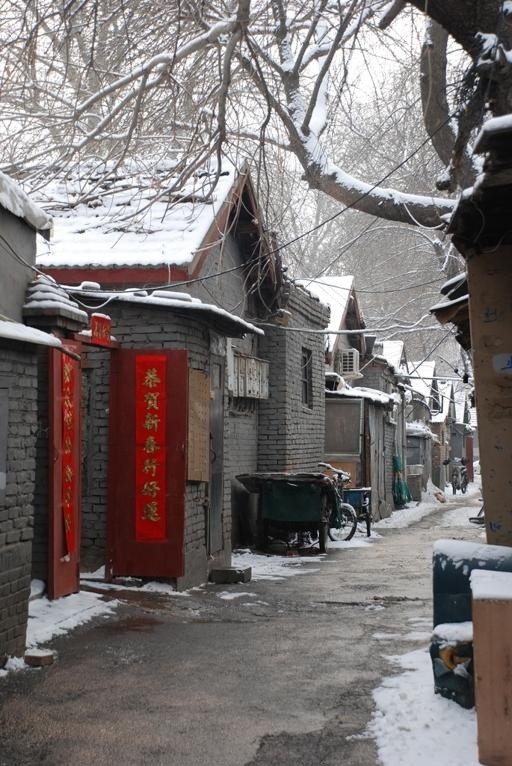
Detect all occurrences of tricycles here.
[254,462,371,553]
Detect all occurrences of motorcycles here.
[442,457,469,494]
